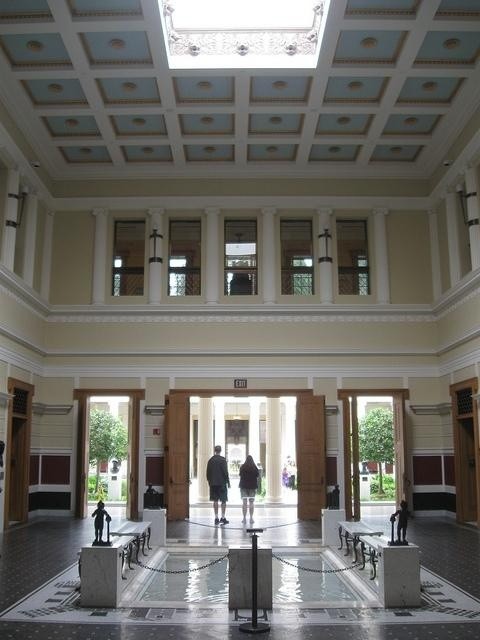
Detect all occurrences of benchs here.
[337,519,386,565]
[77,532,135,591]
[353,529,425,593]
[110,519,155,560]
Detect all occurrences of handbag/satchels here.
[257,477,261,495]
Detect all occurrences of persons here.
[255,462,264,494]
[239,455,260,524]
[110,458,119,472]
[282,455,297,491]
[92,500,110,543]
[206,445,230,525]
[333,484,340,493]
[392,500,412,543]
[146,483,159,494]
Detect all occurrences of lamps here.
[318,222,334,264]
[148,221,163,265]
[5,185,29,229]
[455,182,480,226]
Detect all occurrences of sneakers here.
[215,519,219,525]
[250,519,255,524]
[241,518,246,524]
[220,518,229,524]
